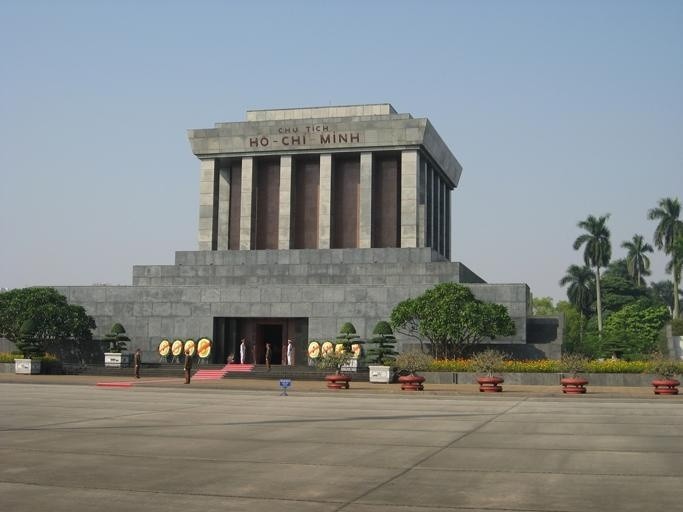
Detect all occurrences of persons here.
[240,339,246,364]
[184,350,191,384]
[134,348,142,379]
[265,343,272,370]
[287,340,294,365]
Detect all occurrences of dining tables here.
[473,348,503,393]
[101,321,132,368]
[391,349,433,391]
[337,321,360,371]
[643,347,682,396]
[12,319,41,376]
[557,349,591,395]
[365,320,397,385]
[316,348,353,391]
